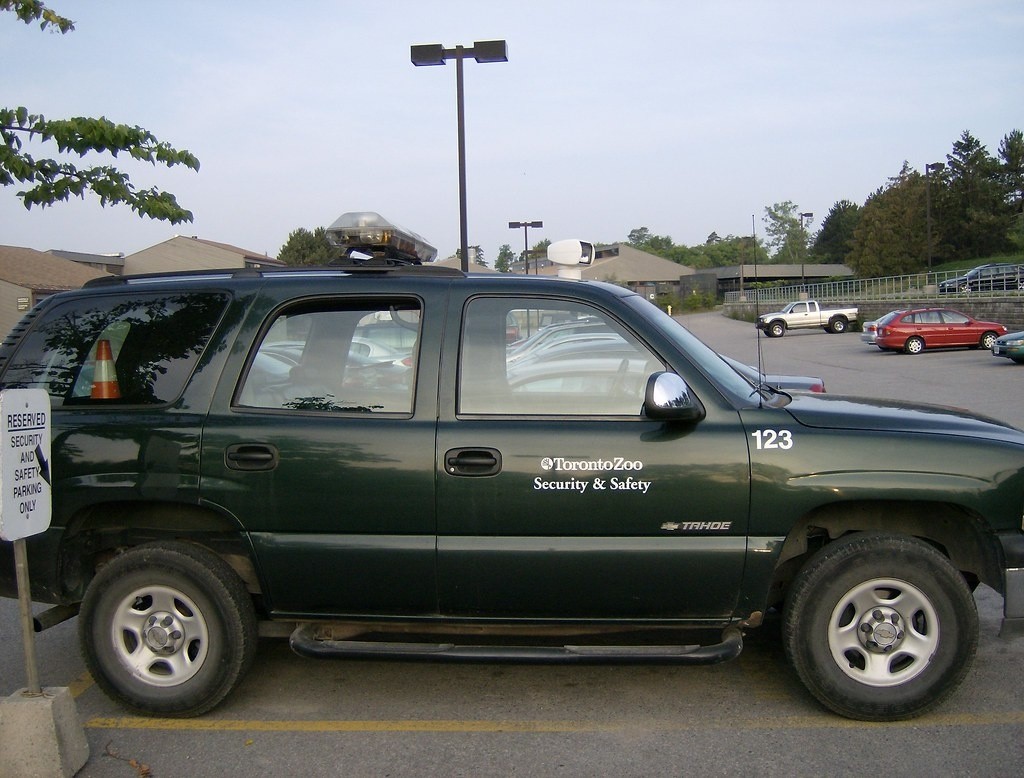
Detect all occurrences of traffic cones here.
[91,340,121,399]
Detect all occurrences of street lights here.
[508,221,543,336]
[801,213,813,285]
[410,39,510,271]
[925,163,945,267]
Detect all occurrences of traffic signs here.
[0,389,52,541]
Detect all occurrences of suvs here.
[0,265,1023,722]
[353,321,419,354]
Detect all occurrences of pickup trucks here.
[755,301,858,338]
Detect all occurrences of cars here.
[250,345,412,392]
[504,311,828,398]
[860,308,953,352]
[991,331,1024,364]
[350,336,415,368]
[939,262,1024,291]
[874,308,1008,355]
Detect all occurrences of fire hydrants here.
[667,305,672,316]
